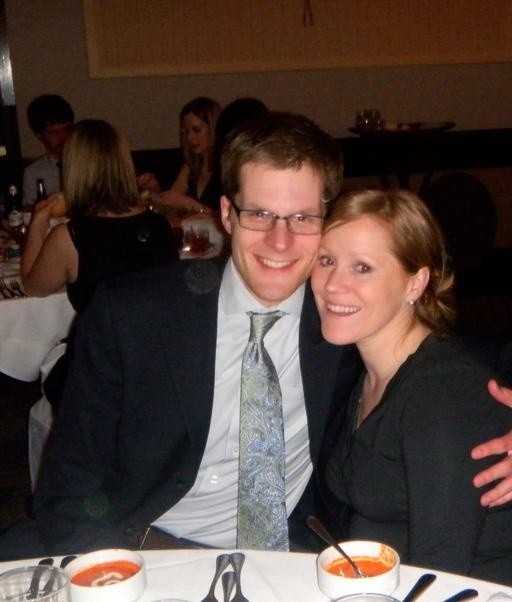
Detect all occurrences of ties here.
[236,309,290,553]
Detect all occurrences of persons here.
[135,94,222,217]
[211,96,271,176]
[310,184,512,589]
[19,93,75,209]
[0,110,512,564]
[17,117,182,490]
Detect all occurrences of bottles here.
[35,178,47,202]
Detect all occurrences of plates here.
[348,120,455,136]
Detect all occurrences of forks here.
[0,279,16,298]
[10,280,28,298]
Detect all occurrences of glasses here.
[232,196,326,235]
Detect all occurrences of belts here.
[130,522,201,549]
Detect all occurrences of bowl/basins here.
[316,540,399,597]
[64,550,146,600]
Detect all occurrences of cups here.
[355,108,381,129]
[189,229,210,256]
[8,210,22,226]
[23,213,31,224]
[0,567,69,602]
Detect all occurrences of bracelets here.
[193,202,208,214]
